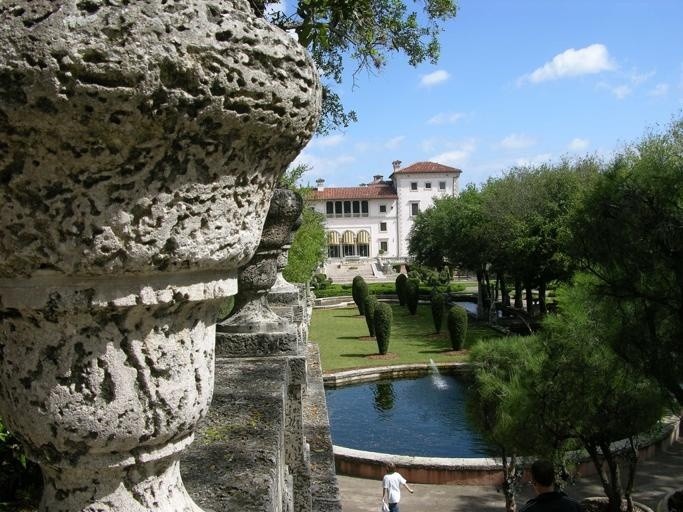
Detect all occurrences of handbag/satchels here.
[382,502,390,512]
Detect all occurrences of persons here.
[381,462,414,512]
[519,460,589,511]
